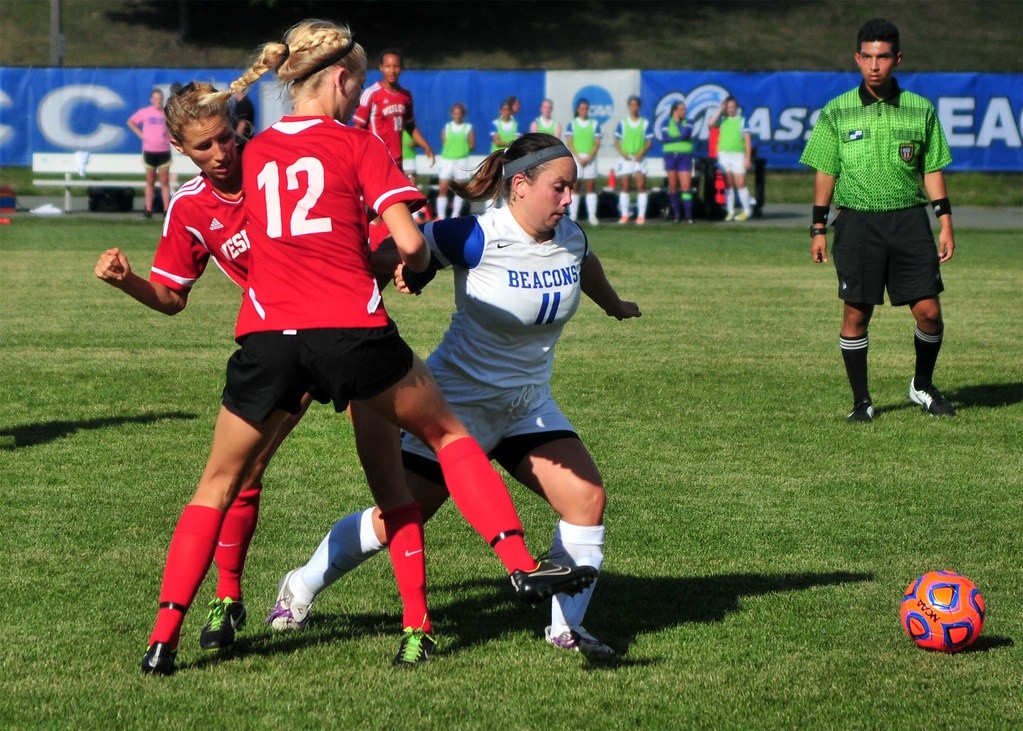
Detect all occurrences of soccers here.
[901,570,985,653]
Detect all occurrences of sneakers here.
[909,377,957,418]
[509,558,598,609]
[271,567,310,632]
[544,625,615,659]
[846,399,876,422]
[142,642,178,676]
[199,598,247,649]
[393,631,436,667]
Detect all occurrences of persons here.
[269,133,642,655]
[529,98,561,140]
[137,19,598,675]
[799,18,956,424]
[564,98,602,225]
[614,96,654,225]
[708,96,752,221]
[229,82,255,154]
[127,89,173,221]
[94,81,439,668]
[485,96,522,212]
[352,52,435,173]
[660,101,694,225]
[433,103,475,222]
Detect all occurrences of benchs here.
[29,152,698,211]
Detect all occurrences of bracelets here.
[813,205,830,225]
[930,197,952,218]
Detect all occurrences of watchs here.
[809,225,827,238]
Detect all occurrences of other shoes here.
[635,216,646,226]
[143,211,153,220]
[724,211,749,222]
[589,216,599,225]
[673,216,680,223]
[162,212,167,219]
[685,218,694,224]
[619,216,630,224]
